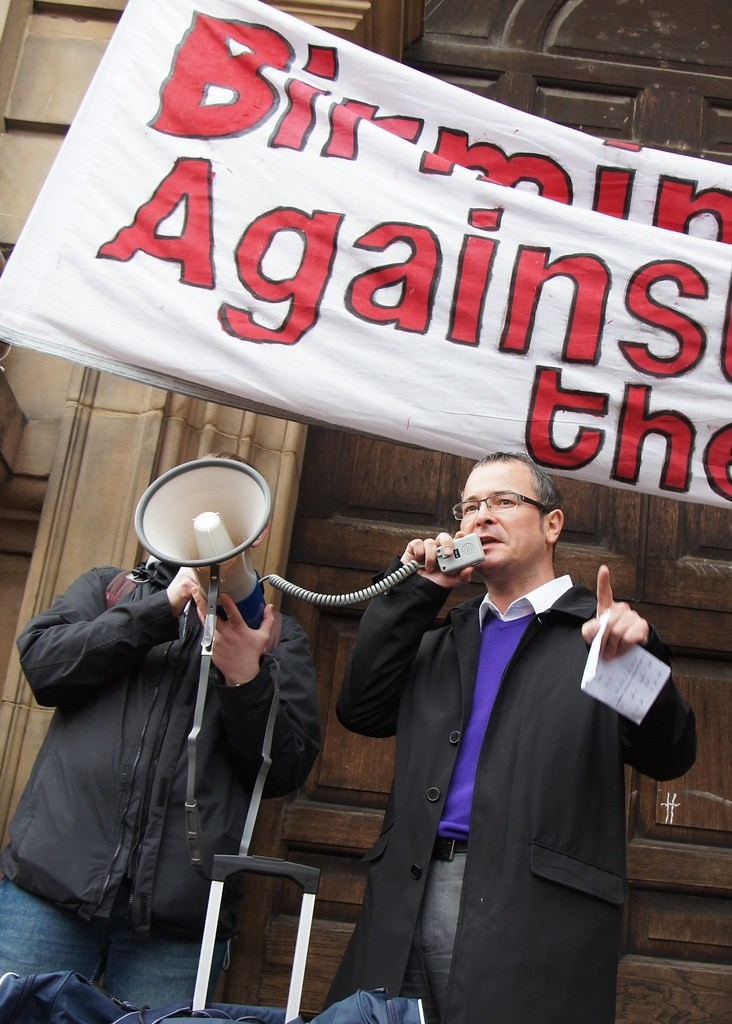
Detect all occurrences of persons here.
[323,451,698,1024]
[0,452,322,1024]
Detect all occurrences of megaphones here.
[135,458,272,629]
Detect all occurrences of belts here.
[430,836,468,862]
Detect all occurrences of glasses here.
[452,492,550,520]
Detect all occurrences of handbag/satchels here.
[0,970,425,1024]
